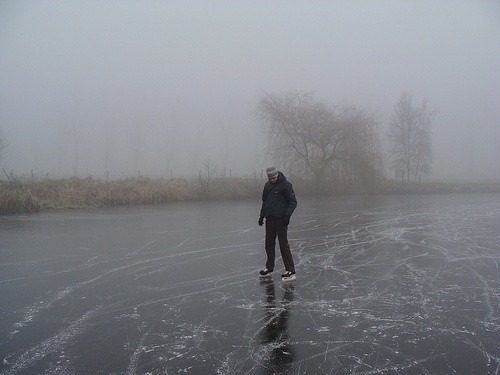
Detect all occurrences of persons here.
[258,166,297,282]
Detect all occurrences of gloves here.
[258,216,264,226]
[283,215,289,226]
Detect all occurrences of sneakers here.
[260,268,273,277]
[281,271,296,281]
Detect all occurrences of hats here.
[266,167,279,179]
